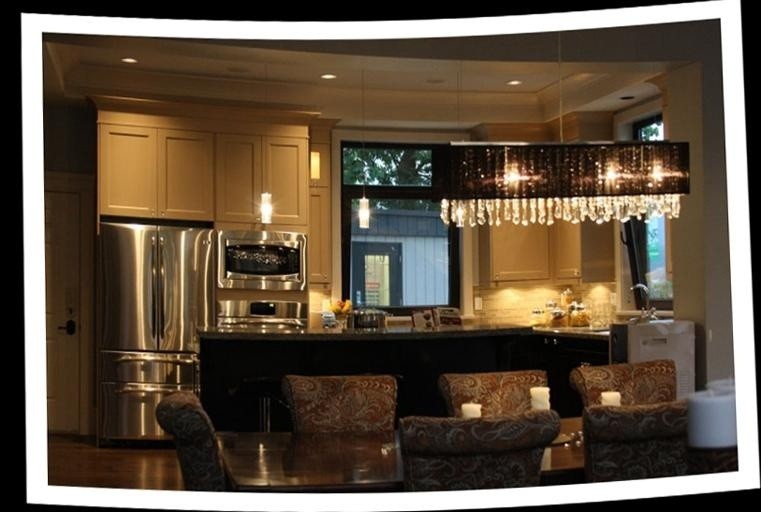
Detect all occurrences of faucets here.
[630,284,649,310]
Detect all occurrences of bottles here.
[530,302,590,329]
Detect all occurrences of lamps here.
[354,70,371,230]
[439,34,690,228]
[258,61,272,224]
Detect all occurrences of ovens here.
[217,299,308,335]
[216,233,307,294]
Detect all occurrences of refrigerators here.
[97,216,215,450]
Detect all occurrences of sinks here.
[584,330,611,335]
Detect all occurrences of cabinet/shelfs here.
[464,110,615,288]
[94,111,217,221]
[215,122,312,230]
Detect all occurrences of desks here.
[213,415,583,495]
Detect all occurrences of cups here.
[684,378,736,451]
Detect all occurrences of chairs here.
[566,360,677,406]
[282,371,397,429]
[398,410,563,490]
[155,387,239,491]
[583,399,693,484]
[441,370,553,419]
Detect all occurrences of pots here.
[346,309,388,329]
